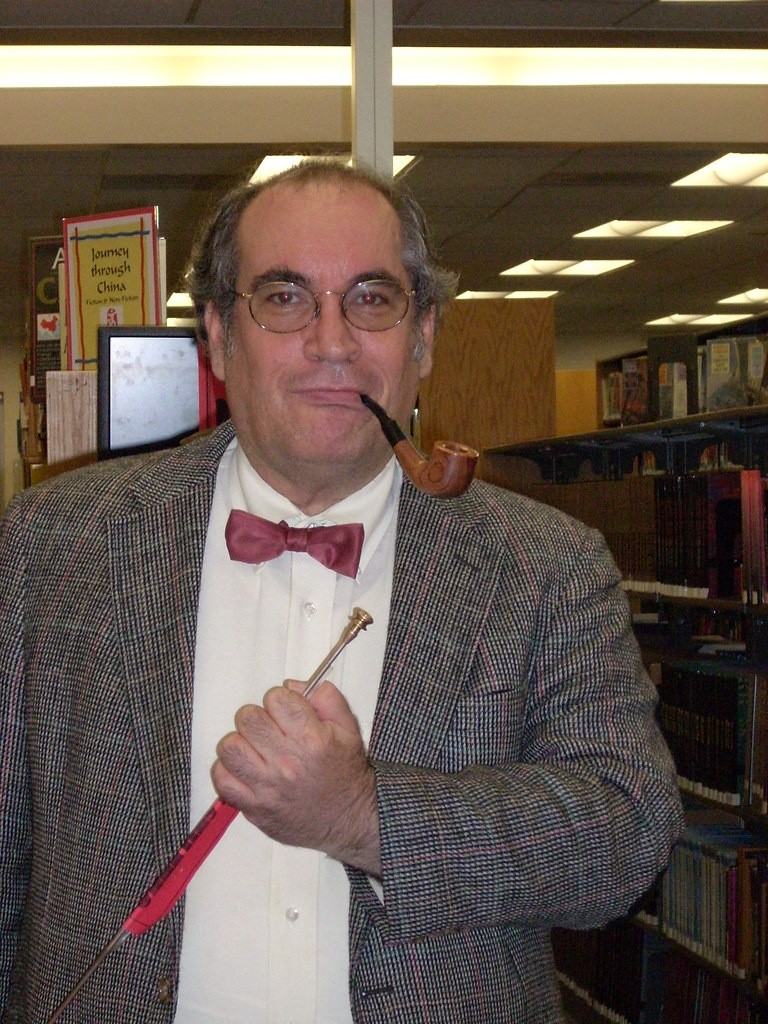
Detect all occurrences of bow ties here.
[222,506,367,583]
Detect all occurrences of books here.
[597,326,768,996]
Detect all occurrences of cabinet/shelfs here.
[480,407,768,1024]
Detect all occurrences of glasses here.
[223,277,420,334]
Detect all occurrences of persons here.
[1,158,683,1024]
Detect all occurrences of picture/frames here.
[97,325,199,461]
[28,234,66,403]
